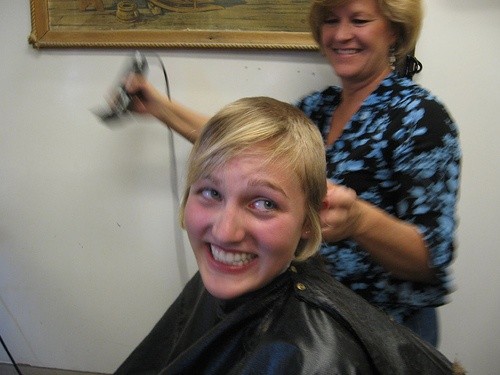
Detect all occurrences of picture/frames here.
[28,0,321,51]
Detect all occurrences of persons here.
[124,0,462,348]
[114,97,467,375]
